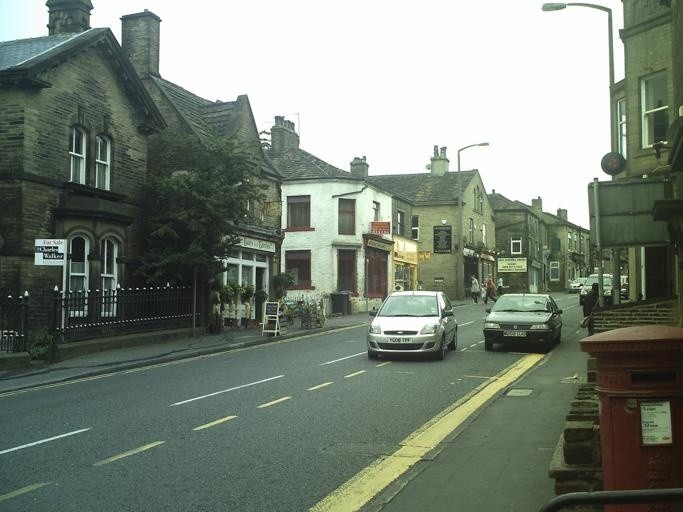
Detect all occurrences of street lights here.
[542,2,621,305]
[457,143,490,299]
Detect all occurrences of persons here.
[581,283,599,337]
[470,272,504,305]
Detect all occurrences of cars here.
[569,273,628,304]
[366,285,457,360]
[483,294,562,351]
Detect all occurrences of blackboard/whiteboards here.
[264,302,279,316]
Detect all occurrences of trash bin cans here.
[580,325,682,511]
[331,290,353,316]
